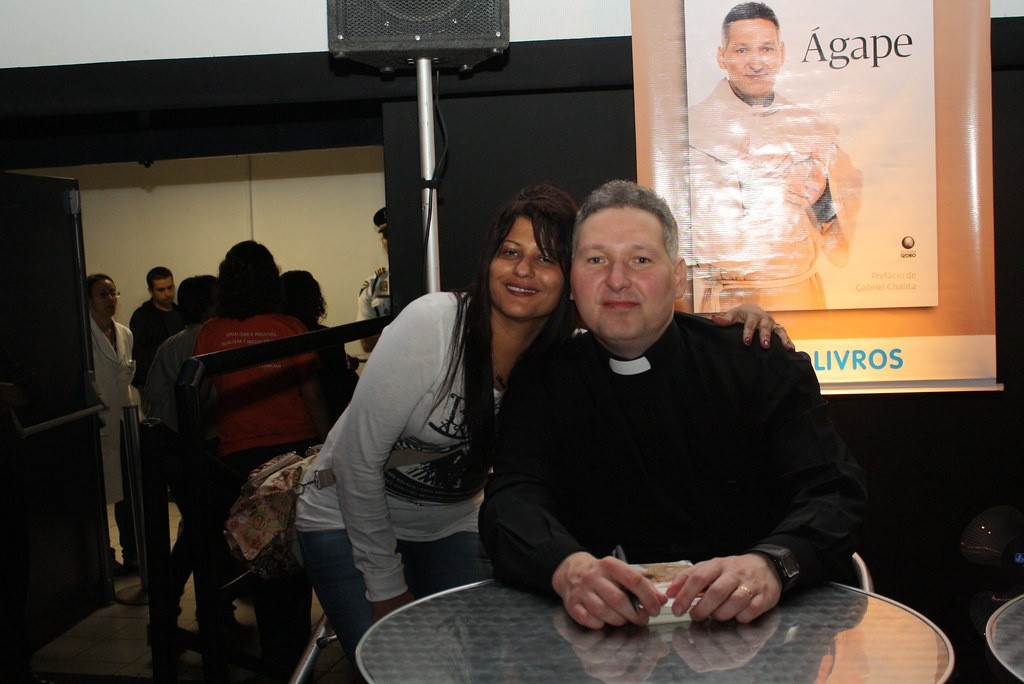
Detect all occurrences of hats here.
[373,207,387,227]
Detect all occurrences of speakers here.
[326,0,510,76]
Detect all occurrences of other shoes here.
[231,612,253,639]
[177,627,196,640]
[114,561,136,576]
[110,547,122,569]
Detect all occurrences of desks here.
[986,594,1024,684]
[355,575,956,684]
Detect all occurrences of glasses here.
[379,227,387,239]
[91,292,120,299]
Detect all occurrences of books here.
[628,559,704,625]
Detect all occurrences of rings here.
[737,583,754,598]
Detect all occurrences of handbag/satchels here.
[223,450,307,582]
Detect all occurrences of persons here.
[275,270,357,420]
[356,208,390,353]
[191,240,330,684]
[131,267,184,383]
[293,183,796,684]
[691,2,864,312]
[477,178,872,630]
[143,275,254,650]
[87,274,142,576]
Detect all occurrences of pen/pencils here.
[613,544,642,617]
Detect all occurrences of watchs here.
[742,543,802,606]
